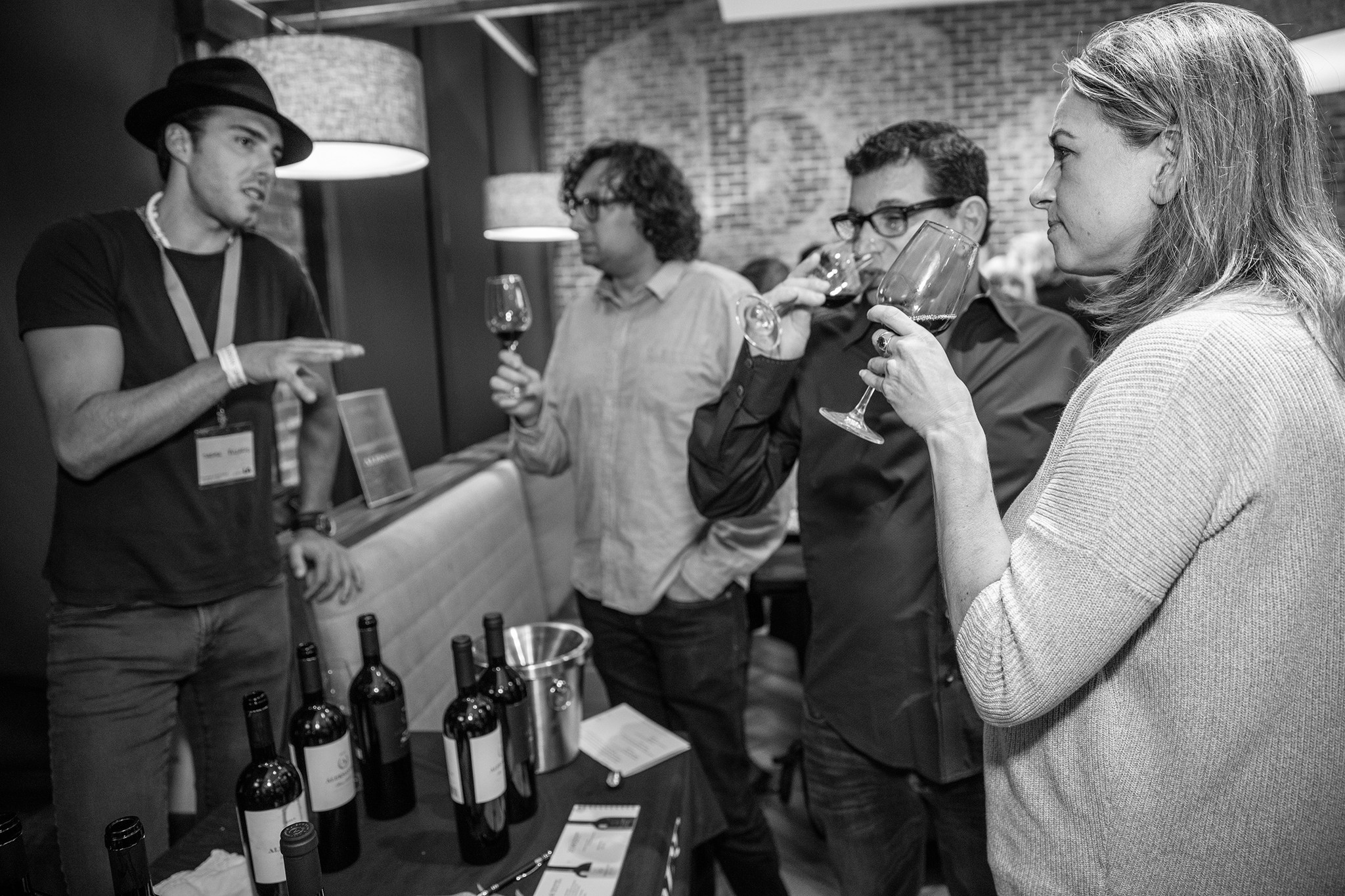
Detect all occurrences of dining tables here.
[114,727,732,896]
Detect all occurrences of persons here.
[685,118,1093,894]
[486,138,790,896]
[16,58,369,895]
[856,2,1345,895]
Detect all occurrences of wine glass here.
[817,220,978,444]
[484,271,532,352]
[735,240,884,352]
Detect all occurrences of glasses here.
[831,196,961,244]
[565,194,635,222]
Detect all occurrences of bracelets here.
[213,344,248,391]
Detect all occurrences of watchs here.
[290,510,338,537]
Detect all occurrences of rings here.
[876,331,895,357]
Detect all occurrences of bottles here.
[289,641,364,875]
[349,612,419,819]
[101,687,323,896]
[446,630,513,868]
[482,612,541,825]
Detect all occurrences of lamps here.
[213,2,432,184]
[484,8,584,243]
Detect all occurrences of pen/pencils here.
[477,849,553,896]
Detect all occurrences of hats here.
[123,57,313,167]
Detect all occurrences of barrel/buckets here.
[468,622,593,775]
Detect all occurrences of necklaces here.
[144,190,174,251]
